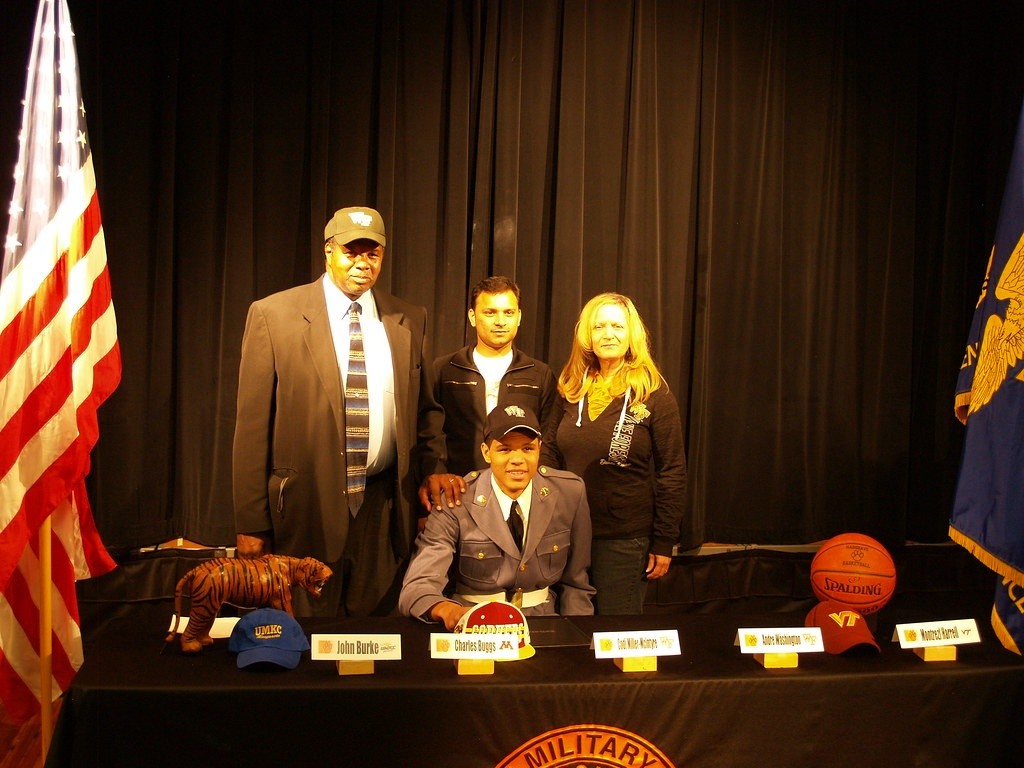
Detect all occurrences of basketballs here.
[810,532,897,616]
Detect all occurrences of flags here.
[0,0,123,767]
[948,100,1022,656]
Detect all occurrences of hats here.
[454,599,537,662]
[227,608,310,669]
[325,206,387,246]
[482,402,541,443]
[805,601,881,654]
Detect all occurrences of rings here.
[449,478,455,483]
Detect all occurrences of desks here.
[47,595,1024,767]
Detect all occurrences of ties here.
[345,303,369,519]
[507,501,525,552]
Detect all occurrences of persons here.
[397,402,596,633]
[232,206,467,617]
[434,276,553,476]
[538,292,687,614]
